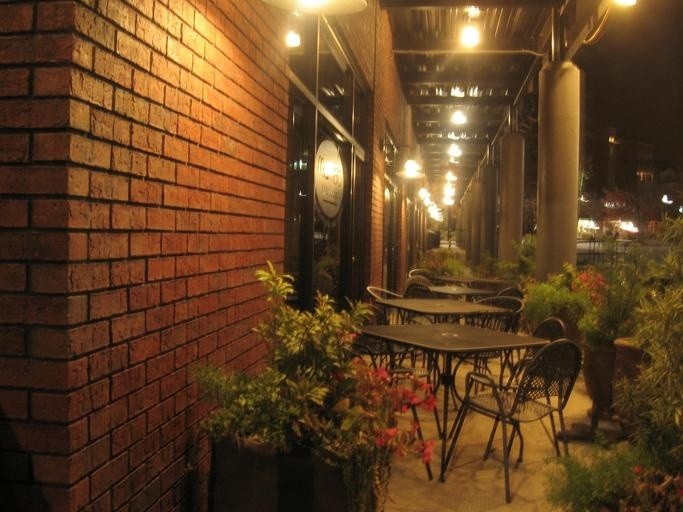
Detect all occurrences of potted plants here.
[577,210,683,424]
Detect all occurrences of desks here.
[359,324,552,447]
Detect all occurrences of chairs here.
[443,337,576,502]
[353,268,525,413]
[461,316,571,462]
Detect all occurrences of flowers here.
[191,258,440,511]
